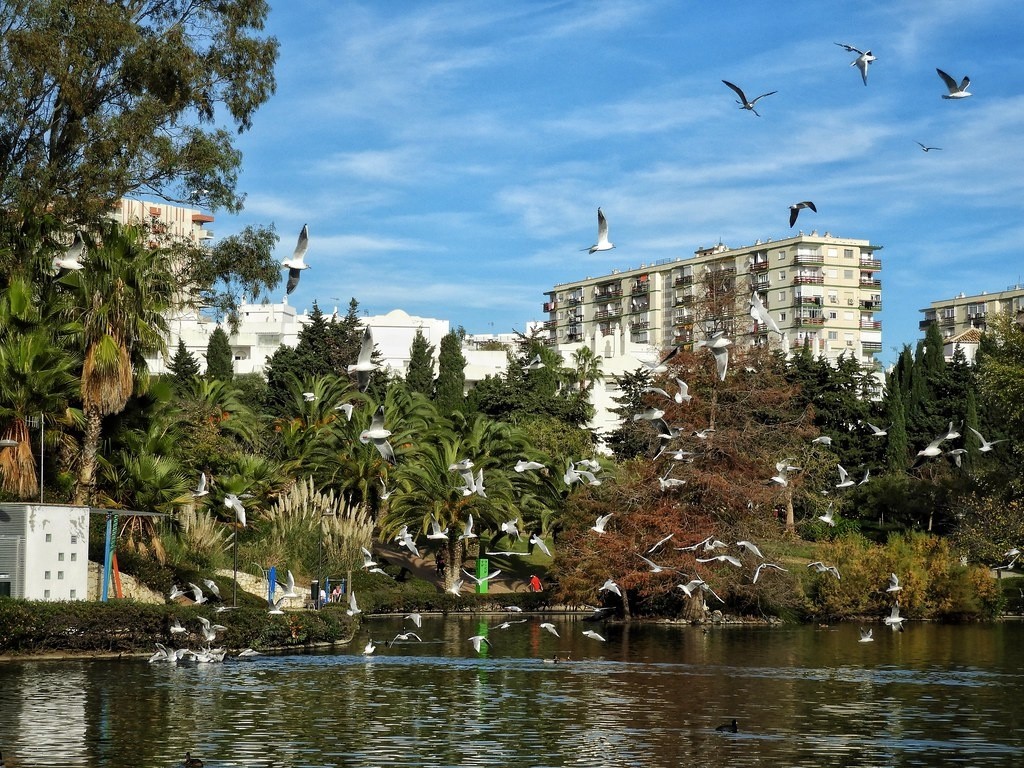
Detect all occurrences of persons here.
[527,574,543,592]
[435,550,446,576]
[316,585,342,610]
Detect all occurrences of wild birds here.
[936,68,972,100]
[50,231,86,270]
[580,206,617,255]
[857,570,909,643]
[768,420,1011,528]
[147,579,264,663]
[722,79,778,118]
[346,458,621,657]
[303,323,397,465]
[634,532,841,604]
[992,548,1022,573]
[184,473,248,529]
[632,286,783,494]
[834,40,878,87]
[280,224,312,294]
[912,138,943,153]
[266,570,302,615]
[788,201,818,229]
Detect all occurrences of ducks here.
[184,752,203,768]
[716,718,740,734]
[540,654,572,664]
